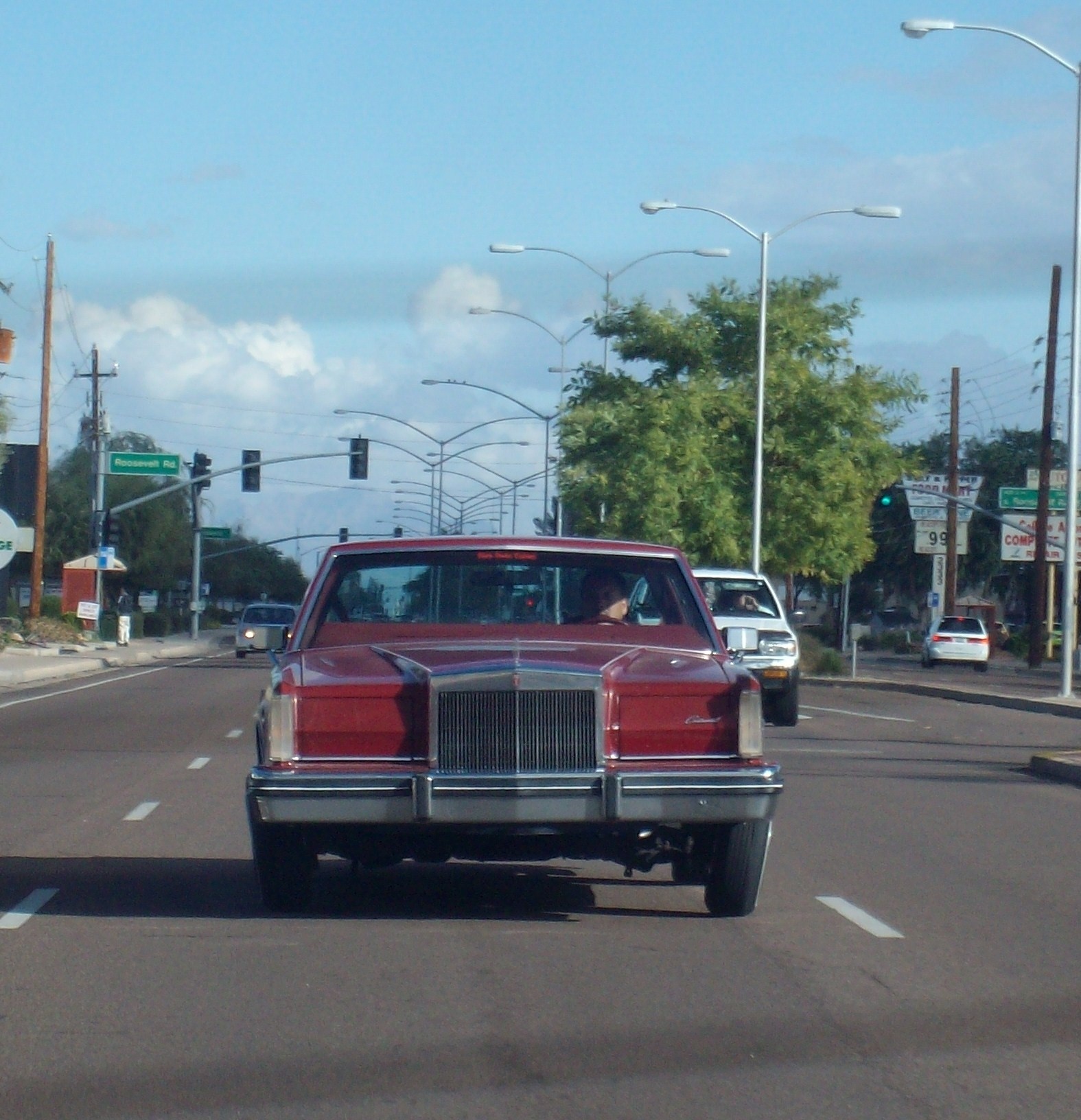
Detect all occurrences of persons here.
[116,584,133,647]
[729,590,775,616]
[580,565,631,625]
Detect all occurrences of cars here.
[920,616,989,674]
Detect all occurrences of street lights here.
[489,245,728,523]
[333,379,579,624]
[902,20,1080,697]
[468,307,661,625]
[641,200,902,576]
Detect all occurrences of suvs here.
[246,540,786,917]
[626,568,806,727]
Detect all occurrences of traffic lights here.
[100,512,120,549]
[881,488,897,526]
[192,452,212,495]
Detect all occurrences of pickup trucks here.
[234,603,294,659]
